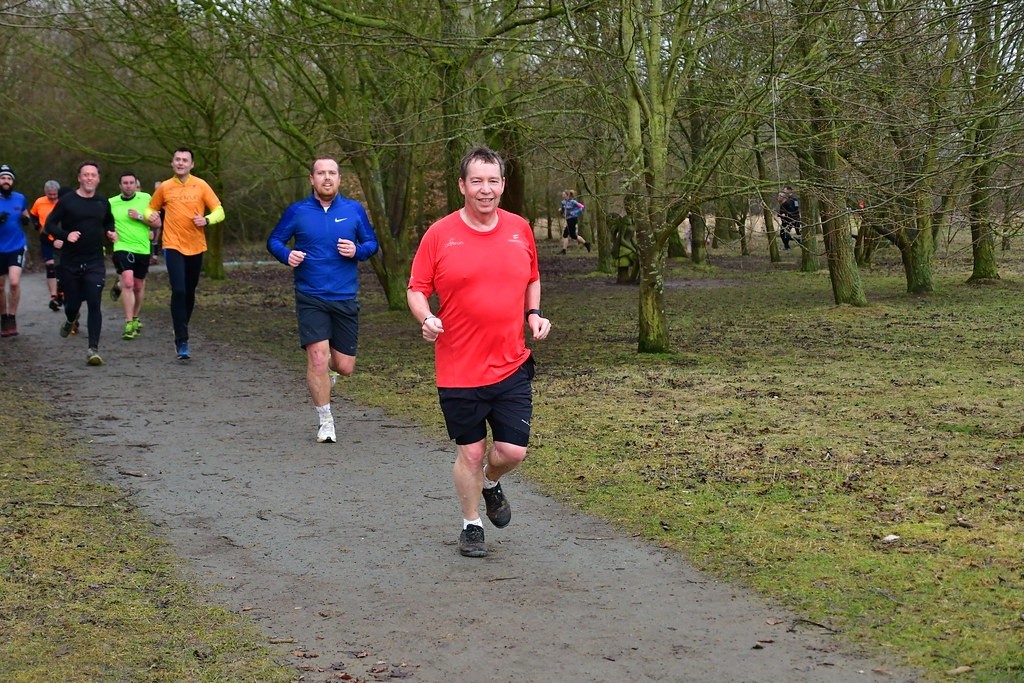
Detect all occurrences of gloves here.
[34,223,39,231]
[19,217,29,226]
[0,213,10,225]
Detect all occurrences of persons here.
[557,190,591,255]
[143,148,225,360]
[29,179,80,335]
[105,172,166,338]
[0,165,30,337]
[777,186,802,250]
[266,155,378,443]
[44,161,117,367]
[406,145,552,558]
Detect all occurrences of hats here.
[0,164,15,181]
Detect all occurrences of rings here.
[347,249,349,252]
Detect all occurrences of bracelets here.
[423,315,435,325]
[205,218,209,226]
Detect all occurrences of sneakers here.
[460,524,487,556]
[176,341,191,359]
[86,348,103,364]
[328,354,337,387]
[316,421,337,442]
[133,317,143,335]
[60,320,74,338]
[122,321,137,338]
[110,275,121,301]
[481,465,511,528]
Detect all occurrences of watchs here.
[137,214,143,220]
[527,309,544,322]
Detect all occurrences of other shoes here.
[556,250,567,255]
[58,294,64,307]
[49,299,59,311]
[0,319,17,336]
[586,242,591,253]
